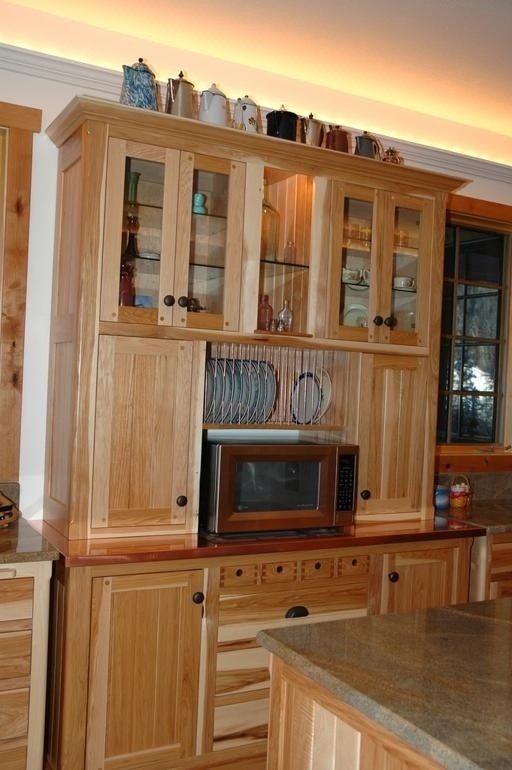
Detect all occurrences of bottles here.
[282,240,296,263]
[192,193,208,214]
[258,293,294,335]
[119,214,141,304]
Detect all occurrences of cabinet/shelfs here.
[297,352,441,527]
[206,528,380,769]
[43,335,209,545]
[332,148,474,358]
[43,81,248,336]
[379,522,491,617]
[26,521,207,769]
[247,131,333,347]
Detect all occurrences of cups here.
[343,223,409,251]
[393,277,416,287]
[343,266,370,288]
[126,171,141,201]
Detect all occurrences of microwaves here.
[200,435,359,535]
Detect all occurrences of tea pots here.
[116,58,402,165]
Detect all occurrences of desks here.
[253,592,512,770]
[1,478,63,768]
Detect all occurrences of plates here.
[341,303,369,327]
[203,358,278,424]
[393,286,416,291]
[290,367,331,425]
[138,252,161,261]
